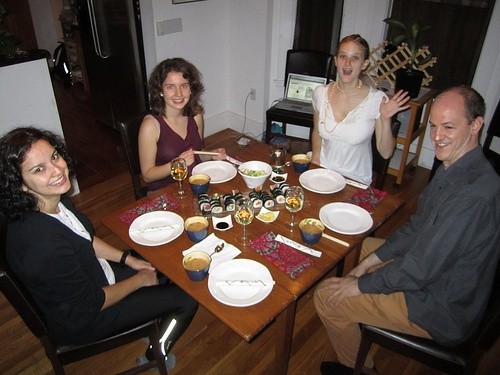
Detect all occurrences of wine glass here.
[271,144,287,174]
[170,158,190,199]
[234,198,255,246]
[284,185,305,232]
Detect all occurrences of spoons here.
[209,242,224,257]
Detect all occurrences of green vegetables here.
[244,169,265,176]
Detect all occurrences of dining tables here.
[97,143,405,374]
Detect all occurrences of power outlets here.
[250,88,255,100]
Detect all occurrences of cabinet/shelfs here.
[58,15,90,95]
[360,76,435,184]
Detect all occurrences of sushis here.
[197,184,290,214]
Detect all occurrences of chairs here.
[0,271,169,375]
[371,119,402,189]
[351,289,500,375]
[482,102,500,175]
[118,112,162,199]
[265,49,333,154]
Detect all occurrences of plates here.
[192,160,238,185]
[299,169,347,194]
[255,207,281,224]
[212,215,233,231]
[128,211,184,246]
[269,172,288,184]
[318,202,373,235]
[208,258,274,307]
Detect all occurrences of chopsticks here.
[322,233,350,247]
[194,151,220,155]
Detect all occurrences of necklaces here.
[324,79,362,134]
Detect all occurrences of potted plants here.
[383,18,428,99]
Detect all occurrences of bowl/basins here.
[299,218,326,245]
[184,216,209,242]
[238,161,272,189]
[182,251,212,281]
[188,173,211,195]
[292,153,312,174]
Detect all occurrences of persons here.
[0,128,199,369]
[314,87,500,375]
[310,34,411,188]
[138,57,226,197]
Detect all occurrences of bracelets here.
[119,250,130,265]
